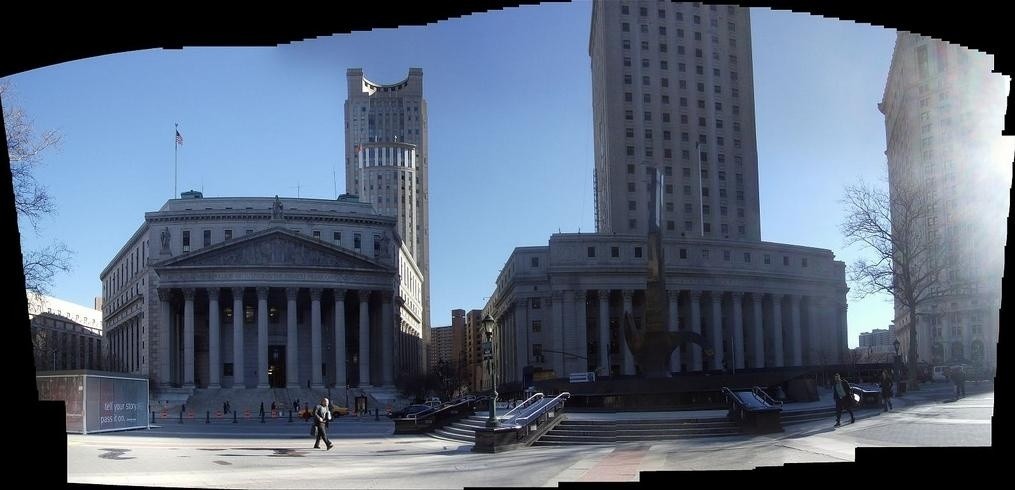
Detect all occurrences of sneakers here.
[313,444,320,449]
[834,420,842,428]
[326,444,334,451]
[850,415,856,423]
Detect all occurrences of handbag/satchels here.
[309,425,319,436]
[849,393,861,407]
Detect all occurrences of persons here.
[832,374,856,426]
[880,370,893,412]
[956,366,967,398]
[313,398,334,450]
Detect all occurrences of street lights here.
[345,384,350,408]
[481,313,499,427]
[52,348,57,371]
[892,336,904,397]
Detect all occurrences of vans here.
[932,365,953,383]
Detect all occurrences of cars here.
[298,404,349,420]
[386,401,441,420]
